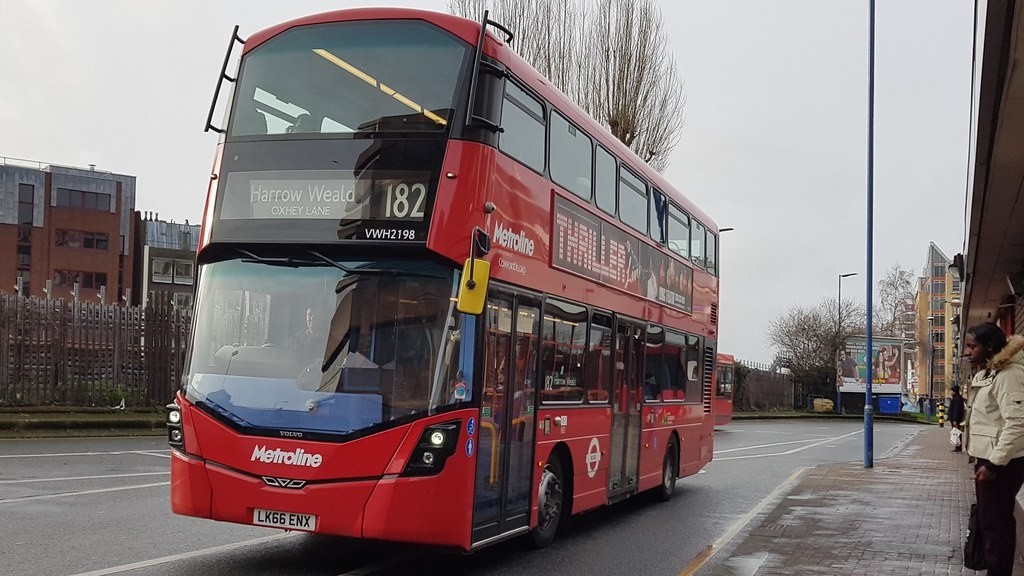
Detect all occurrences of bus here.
[167,8,722,551]
[639,343,734,425]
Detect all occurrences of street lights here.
[927,317,935,422]
[836,273,858,414]
[274,400,288,409]
[221,350,239,390]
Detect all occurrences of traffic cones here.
[841,405,847,415]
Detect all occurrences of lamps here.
[1005,271,1024,297]
[949,253,963,281]
[998,295,1017,307]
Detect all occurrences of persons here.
[284,303,331,354]
[875,347,899,379]
[917,395,926,413]
[498,336,527,435]
[963,322,1024,576]
[947,385,965,452]
[840,349,859,381]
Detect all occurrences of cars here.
[554,372,582,386]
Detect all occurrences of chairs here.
[670,242,716,273]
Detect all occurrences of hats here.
[951,385,960,394]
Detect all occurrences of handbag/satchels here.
[963,504,990,571]
[949,423,962,447]
